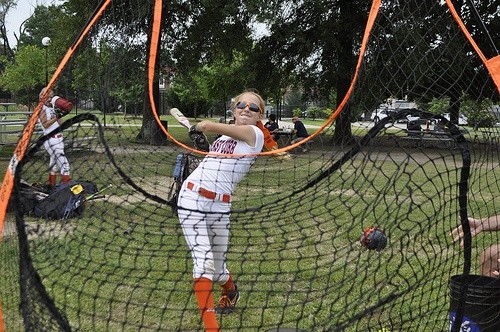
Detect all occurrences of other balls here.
[360,226,387,251]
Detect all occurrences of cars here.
[362,99,469,127]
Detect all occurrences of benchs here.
[401,137,454,148]
[295,140,314,152]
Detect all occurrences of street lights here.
[42,37,53,87]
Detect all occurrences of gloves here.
[188,125,203,140]
[195,134,210,152]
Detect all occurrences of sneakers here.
[215,284,240,316]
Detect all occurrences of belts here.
[187,182,230,202]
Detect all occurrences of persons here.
[448,214,500,278]
[36,88,70,185]
[229,116,236,124]
[267,114,279,138]
[291,117,310,152]
[176,91,264,332]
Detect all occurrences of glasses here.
[236,102,261,115]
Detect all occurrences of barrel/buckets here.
[448,274,500,332]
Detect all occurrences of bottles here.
[50,96,73,112]
[173,152,183,177]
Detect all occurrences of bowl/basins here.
[286,129,291,131]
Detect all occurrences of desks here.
[62,136,97,150]
[270,131,294,156]
[409,131,447,135]
[481,130,500,138]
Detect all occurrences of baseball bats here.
[19,178,113,212]
[170,107,195,132]
